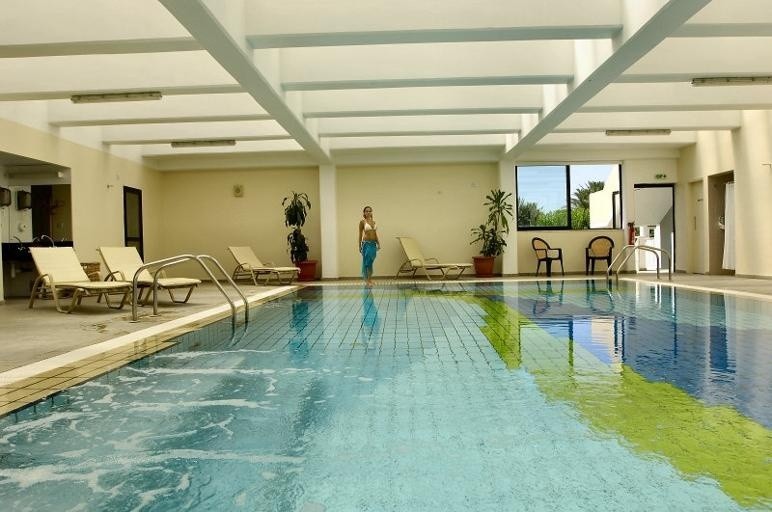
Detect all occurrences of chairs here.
[531,237,564,277]
[97,246,202,309]
[586,280,614,315]
[29,247,132,314]
[394,237,473,280]
[533,281,564,316]
[585,236,614,276]
[397,283,466,316]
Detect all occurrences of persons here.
[359,206,380,288]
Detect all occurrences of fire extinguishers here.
[628,221,635,245]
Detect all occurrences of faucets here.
[60,237,67,242]
[13,235,22,247]
[32,237,39,243]
[41,235,54,247]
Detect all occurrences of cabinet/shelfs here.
[227,246,301,285]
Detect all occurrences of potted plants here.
[470,189,513,277]
[282,191,319,281]
